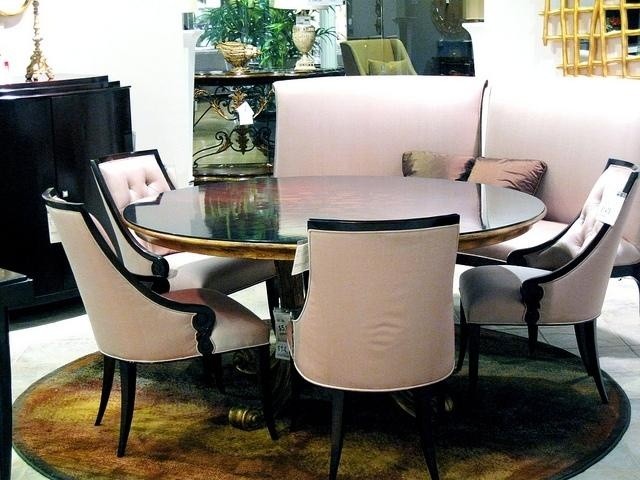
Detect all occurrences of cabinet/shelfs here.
[1,76,134,326]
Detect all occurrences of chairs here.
[39,183,279,460]
[274,213,461,479]
[457,154,639,425]
[338,36,419,75]
[85,148,292,332]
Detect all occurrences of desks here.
[0,267,34,480]
[115,172,549,434]
[193,68,346,181]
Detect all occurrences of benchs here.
[267,75,640,354]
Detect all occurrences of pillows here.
[364,55,411,75]
[465,155,547,198]
[401,148,477,180]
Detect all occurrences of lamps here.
[273,0,329,73]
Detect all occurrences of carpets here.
[14,317,630,479]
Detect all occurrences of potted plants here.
[192,1,272,74]
